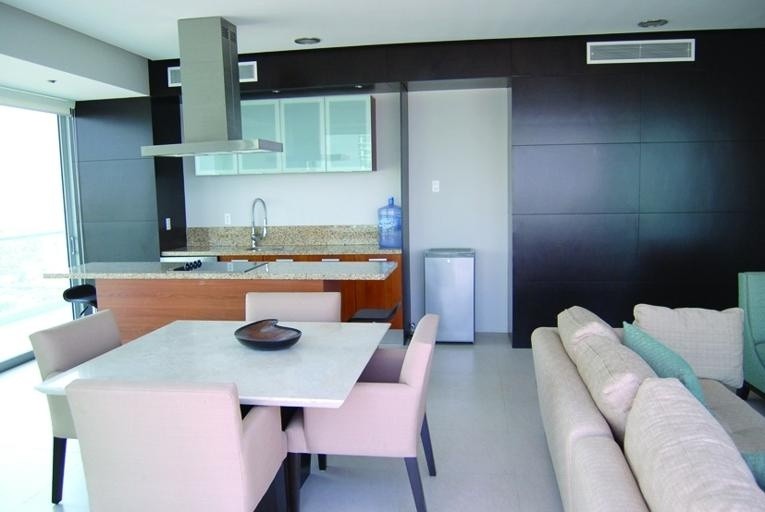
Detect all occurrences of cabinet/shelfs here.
[218,255,405,329]
[194,93,377,176]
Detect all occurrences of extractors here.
[138,17,285,158]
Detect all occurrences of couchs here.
[530,324,765,511]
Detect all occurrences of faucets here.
[250,198,267,248]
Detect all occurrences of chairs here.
[29,308,122,506]
[64,377,287,512]
[736,268,764,400]
[283,312,441,512]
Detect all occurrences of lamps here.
[638,18,669,29]
[295,37,321,45]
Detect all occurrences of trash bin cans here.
[424,247,476,344]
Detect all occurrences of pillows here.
[621,318,704,405]
[623,375,765,512]
[555,305,662,443]
[630,303,745,390]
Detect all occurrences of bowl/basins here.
[234,318,302,349]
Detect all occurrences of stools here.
[63,284,98,315]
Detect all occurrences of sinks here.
[247,248,284,252]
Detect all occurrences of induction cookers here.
[168,260,268,274]
